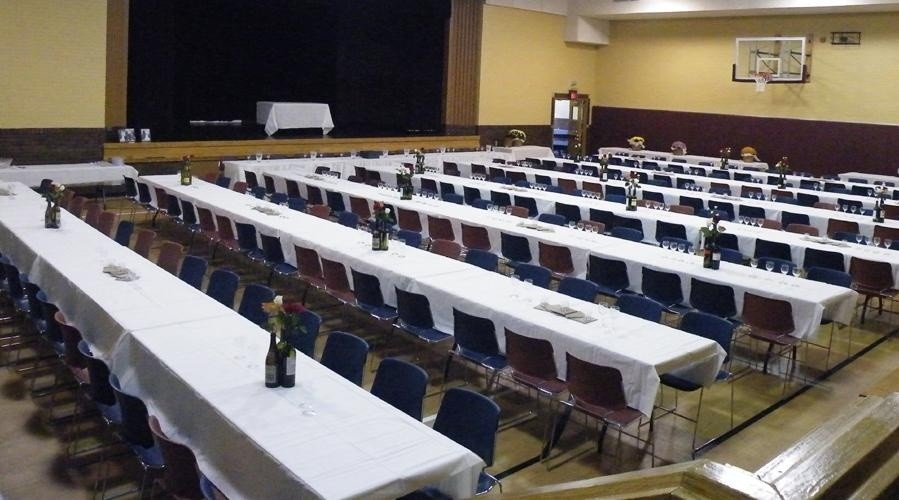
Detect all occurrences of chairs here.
[1,126,898,499]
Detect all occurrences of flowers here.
[775,157,794,173]
[259,289,302,336]
[396,164,414,183]
[872,184,889,201]
[598,151,612,166]
[570,141,584,153]
[671,141,687,149]
[701,211,721,242]
[362,198,398,231]
[626,136,645,145]
[719,143,732,156]
[412,147,425,164]
[622,169,642,190]
[507,129,526,141]
[740,146,757,156]
[181,151,194,170]
[39,178,68,204]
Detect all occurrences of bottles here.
[703,238,721,270]
[625,185,638,212]
[276,327,297,388]
[400,175,413,200]
[873,199,885,222]
[415,157,425,174]
[645,199,671,214]
[720,156,730,170]
[180,160,192,185]
[265,333,280,386]
[778,172,787,189]
[371,210,389,250]
[44,199,61,228]
[599,164,608,182]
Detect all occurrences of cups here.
[243,190,290,220]
[356,224,370,247]
[390,236,407,258]
[246,144,495,164]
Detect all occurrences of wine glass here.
[415,189,440,202]
[322,170,339,184]
[569,220,599,238]
[662,240,697,265]
[580,189,602,201]
[378,182,399,196]
[867,189,879,198]
[486,204,513,223]
[747,192,778,205]
[612,149,639,182]
[833,203,866,218]
[423,165,440,176]
[855,234,892,256]
[509,273,533,304]
[528,181,548,192]
[596,301,621,331]
[750,175,761,184]
[811,182,824,194]
[683,168,702,193]
[468,171,487,182]
[513,152,594,177]
[737,216,765,231]
[750,259,802,287]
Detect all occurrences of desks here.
[256,101,335,136]
